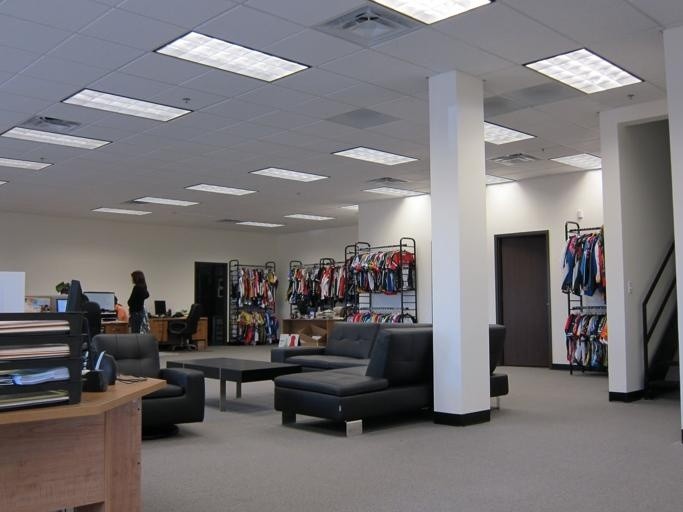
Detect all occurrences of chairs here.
[80,302,100,334]
[169,304,201,351]
[89,334,204,440]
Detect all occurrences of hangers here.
[571,305,607,315]
[568,226,602,237]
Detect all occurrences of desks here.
[281,318,336,346]
[0,375,167,512]
[101,321,129,334]
[148,317,208,346]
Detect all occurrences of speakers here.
[94,350,117,384]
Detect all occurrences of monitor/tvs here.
[154,300,166,317]
[56,299,67,312]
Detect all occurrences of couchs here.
[267,316,511,426]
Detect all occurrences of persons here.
[114,296,127,321]
[127,270,150,333]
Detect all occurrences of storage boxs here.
[293,323,327,346]
